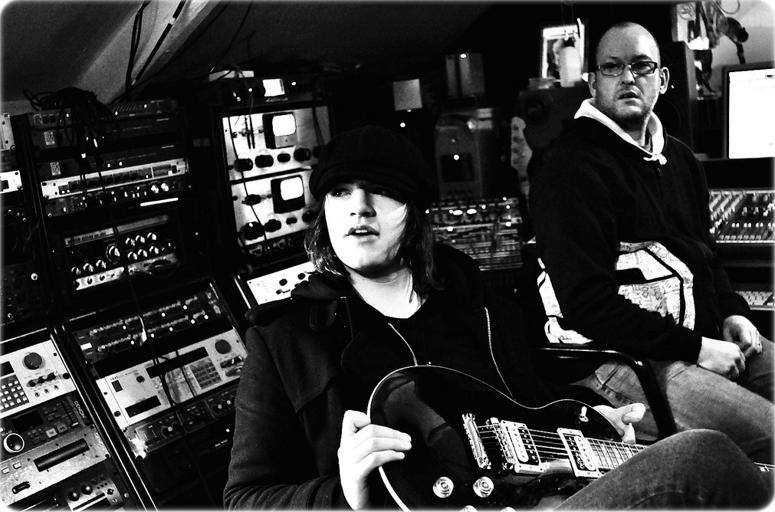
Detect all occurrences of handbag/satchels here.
[528,236,699,355]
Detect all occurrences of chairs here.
[490,234,678,445]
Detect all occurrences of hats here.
[308,123,439,213]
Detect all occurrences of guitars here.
[366,365,772,508]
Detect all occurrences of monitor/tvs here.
[722,60,775,159]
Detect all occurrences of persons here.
[527,20,775,465]
[223,120,774,512]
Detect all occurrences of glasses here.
[592,60,666,77]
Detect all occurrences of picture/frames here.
[540,23,585,83]
